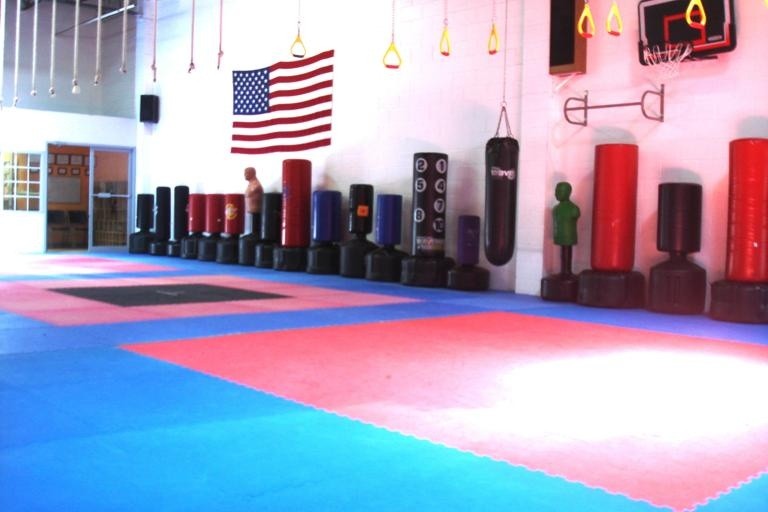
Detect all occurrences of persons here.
[551,181,580,247]
[243,167,264,213]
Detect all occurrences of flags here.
[231,49,335,155]
